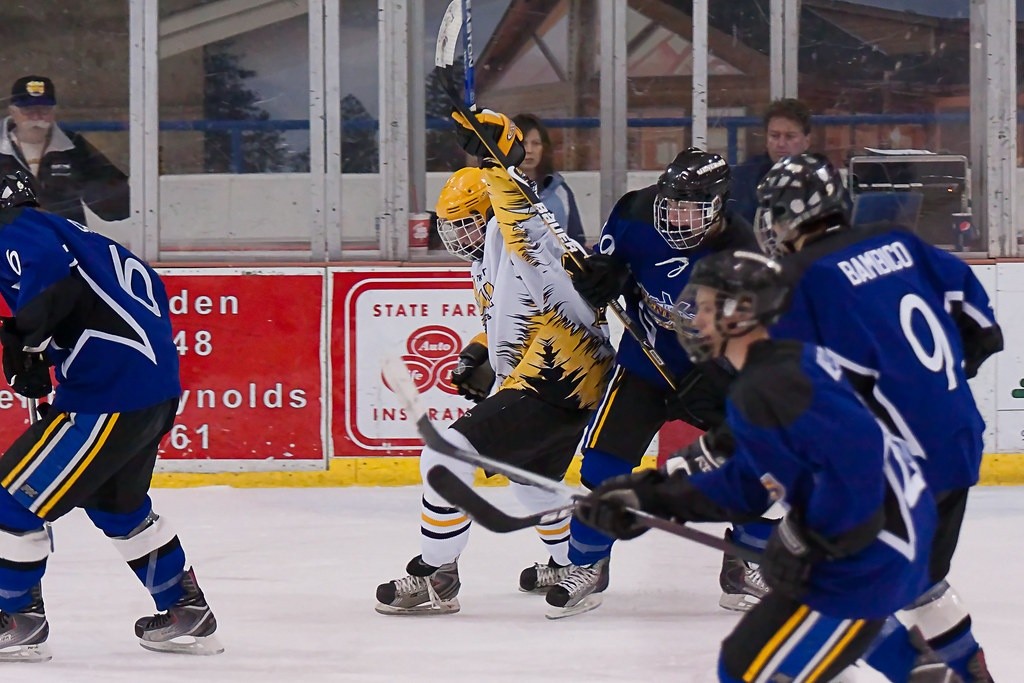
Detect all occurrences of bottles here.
[375,212,381,246]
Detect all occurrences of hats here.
[10,75,57,105]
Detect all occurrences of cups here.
[408,212,430,256]
[952,212,972,251]
[426,210,442,248]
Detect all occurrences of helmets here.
[753,151,852,261]
[654,147,731,251]
[0,167,39,207]
[437,167,495,263]
[672,249,792,367]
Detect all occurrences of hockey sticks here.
[25,391,56,552]
[377,346,763,562]
[434,0,684,403]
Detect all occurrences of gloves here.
[759,509,823,600]
[450,334,496,402]
[3,346,54,398]
[575,471,693,539]
[573,257,624,309]
[452,109,526,166]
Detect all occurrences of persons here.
[376,103,618,608]
[574,248,968,683]
[545,153,1005,683]
[510,113,586,244]
[0,169,218,647]
[724,98,853,216]
[0,76,130,227]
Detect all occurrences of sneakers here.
[518,561,578,594]
[0,610,56,663]
[542,558,611,620]
[717,554,770,611]
[372,563,465,618]
[135,567,226,657]
